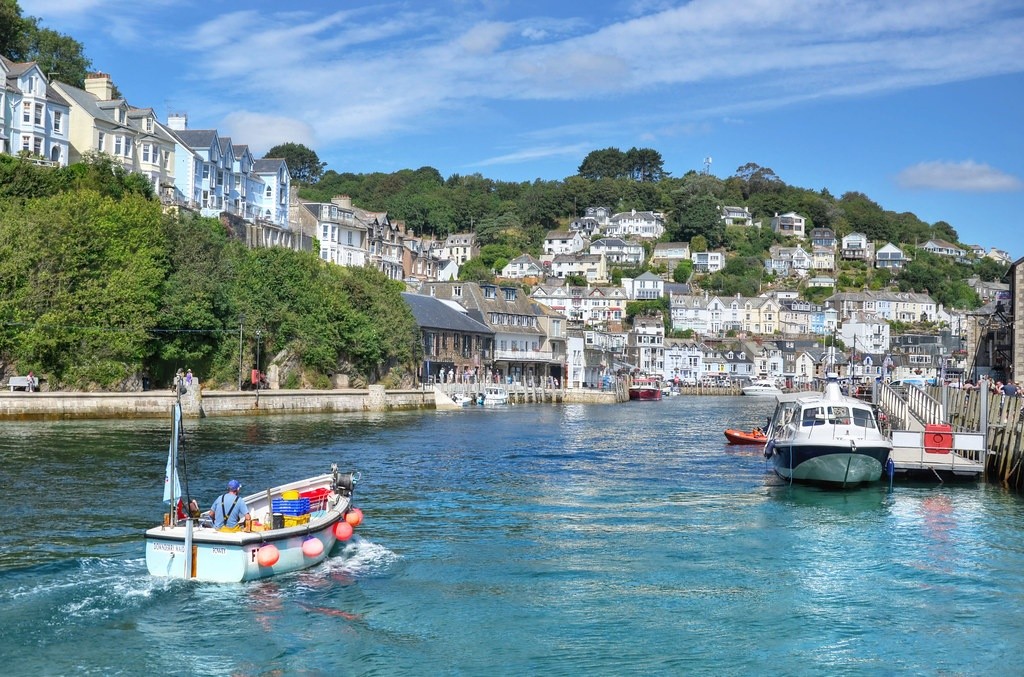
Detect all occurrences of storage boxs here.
[299,487,333,512]
[283,513,311,528]
[271,498,310,517]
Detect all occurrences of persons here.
[418,360,559,393]
[26,370,35,393]
[762,416,771,434]
[260,371,271,389]
[943,371,1024,412]
[186,369,194,385]
[208,480,251,531]
[176,367,185,386]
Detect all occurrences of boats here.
[763,341,893,491]
[725,428,766,445]
[742,381,783,396]
[452,394,473,407]
[143,375,362,583]
[484,387,509,405]
[852,383,873,403]
[629,378,662,401]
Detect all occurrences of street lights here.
[256,327,261,406]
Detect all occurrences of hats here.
[228,480,242,491]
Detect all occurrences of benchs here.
[6,376,39,392]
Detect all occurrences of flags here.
[160,403,183,511]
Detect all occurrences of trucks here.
[888,378,936,387]
